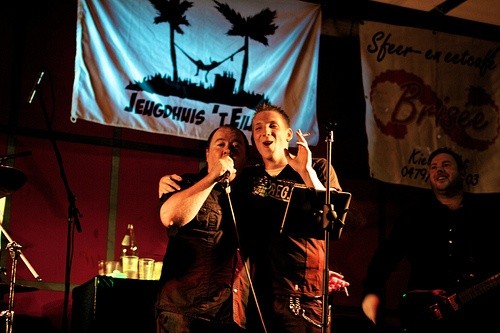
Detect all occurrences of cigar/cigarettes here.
[303,133,310,136]
[343,286,349,296]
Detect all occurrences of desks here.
[71,275,159,333]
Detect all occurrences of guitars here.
[379,271,500,333]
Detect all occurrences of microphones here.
[222,153,232,179]
[28,71,46,103]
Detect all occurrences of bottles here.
[121,223,138,255]
[98,260,122,275]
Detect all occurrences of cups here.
[155,262,163,280]
[138,258,155,280]
[122,256,139,279]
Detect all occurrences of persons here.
[361,148,500,333]
[157,103,342,333]
[158,125,349,333]
